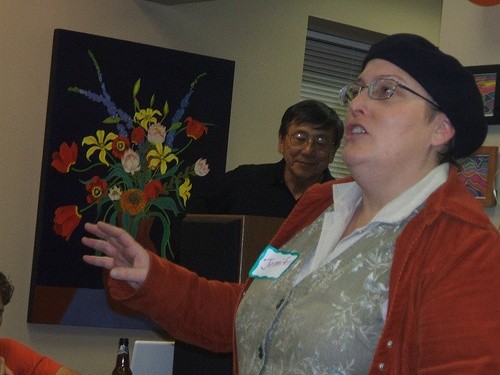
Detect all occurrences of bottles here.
[111,338,133,375]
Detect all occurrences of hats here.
[362,32,488,158]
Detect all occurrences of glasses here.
[337,78,442,111]
[287,132,334,151]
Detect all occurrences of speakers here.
[171,213,288,375]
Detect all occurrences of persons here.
[0,272,80,375]
[207,100,344,218]
[81,33,500,375]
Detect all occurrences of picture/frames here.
[458,146,498,209]
[463,64,500,125]
